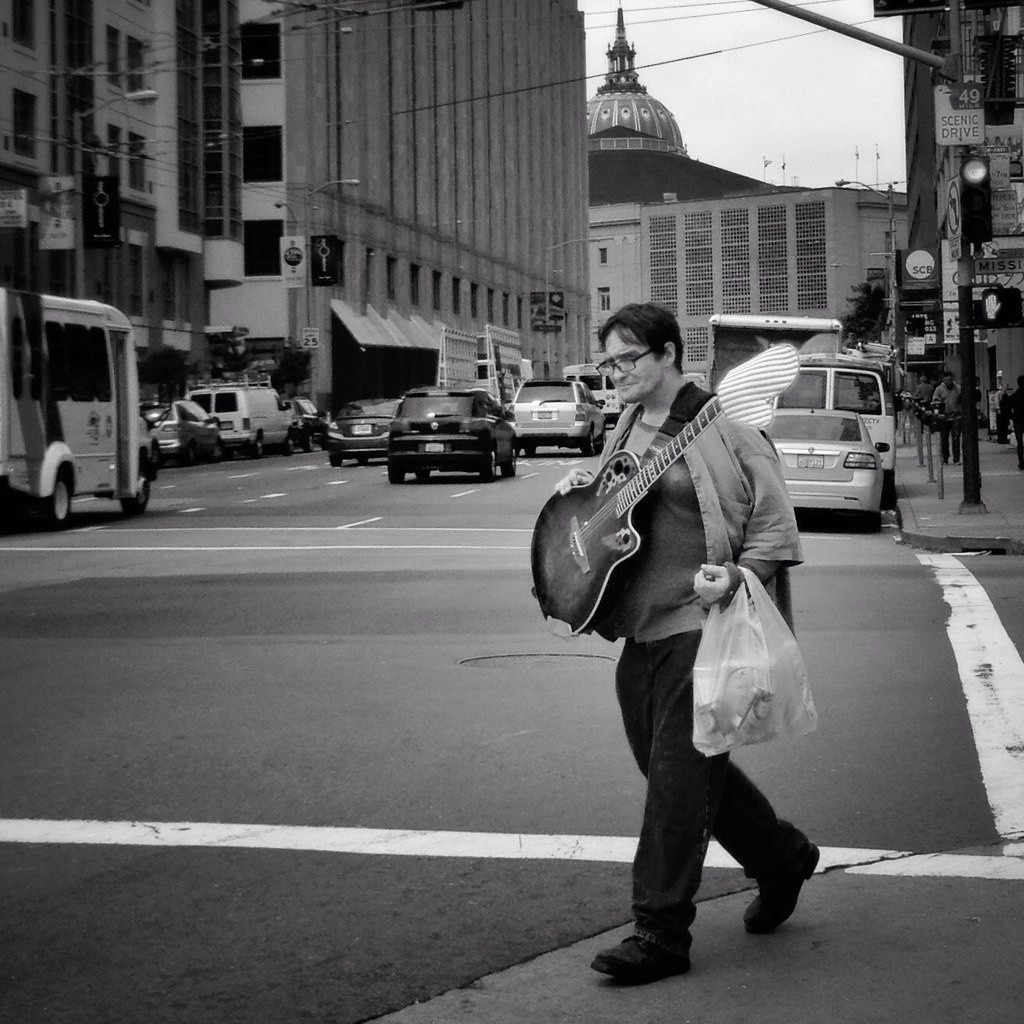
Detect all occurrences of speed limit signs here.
[302,327,321,349]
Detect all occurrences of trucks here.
[475,361,534,406]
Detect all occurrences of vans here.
[773,366,897,506]
[141,401,225,466]
[187,375,296,460]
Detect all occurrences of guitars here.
[530,341,802,640]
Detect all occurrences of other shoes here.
[942,459,948,465]
[953,459,961,465]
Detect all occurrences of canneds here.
[729,685,774,737]
[695,699,737,754]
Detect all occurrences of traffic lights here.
[982,288,1023,326]
[958,155,994,243]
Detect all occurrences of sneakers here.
[591,935,690,984]
[743,838,819,933]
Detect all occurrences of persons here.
[1009,374,1024,471]
[915,375,934,400]
[555,302,821,979]
[931,371,962,465]
[994,383,1011,444]
[975,375,983,442]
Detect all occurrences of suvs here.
[386,390,518,483]
[505,378,605,456]
[283,397,330,452]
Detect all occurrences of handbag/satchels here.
[692,565,816,756]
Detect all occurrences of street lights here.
[273,201,298,394]
[76,90,159,299]
[304,181,361,404]
[835,180,898,361]
[545,237,615,380]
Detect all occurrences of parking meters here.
[898,392,946,501]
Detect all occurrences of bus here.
[706,313,842,401]
[1,288,173,528]
[562,365,627,427]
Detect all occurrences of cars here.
[326,400,402,467]
[767,408,891,532]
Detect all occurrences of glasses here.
[595,349,653,376]
